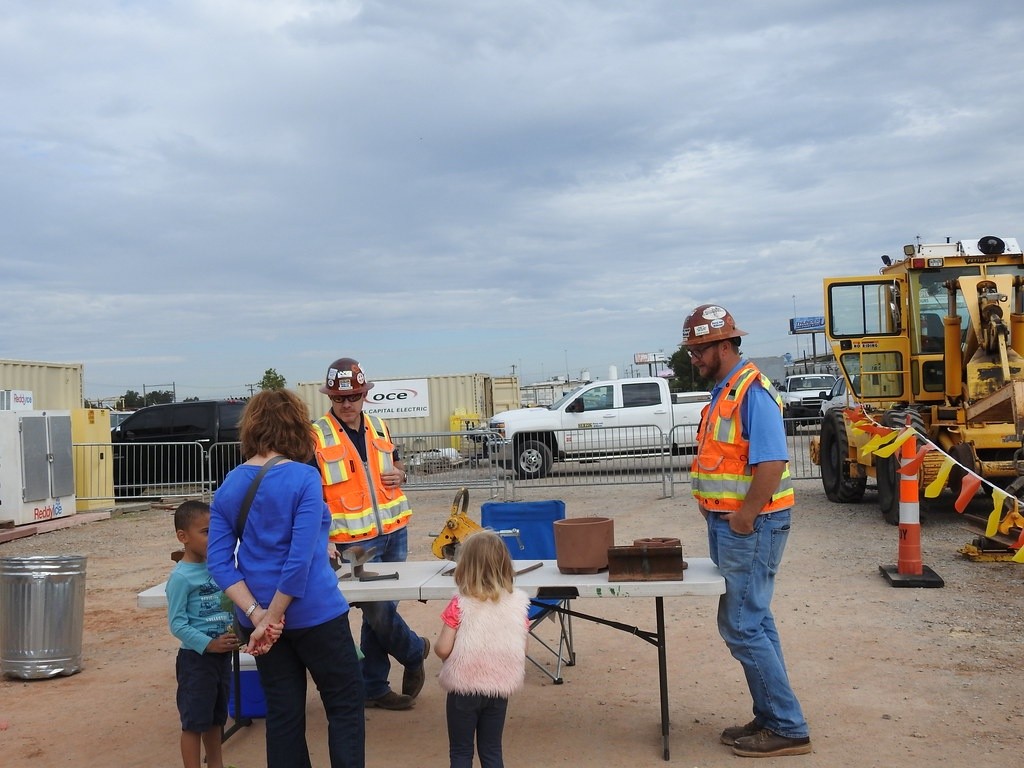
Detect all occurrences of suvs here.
[819,374,860,418]
[775,373,837,436]
[111,401,253,496]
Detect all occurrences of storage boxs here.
[225,652,267,719]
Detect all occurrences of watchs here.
[399,469,407,485]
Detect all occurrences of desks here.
[137,558,727,763]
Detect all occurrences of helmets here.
[676,304,749,347]
[318,358,374,396]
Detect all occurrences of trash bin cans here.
[0,554,87,680]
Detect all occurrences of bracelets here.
[245,601,259,618]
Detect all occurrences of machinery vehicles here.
[811,233,1024,561]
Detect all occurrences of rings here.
[392,480,395,485]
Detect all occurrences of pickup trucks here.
[483,377,714,478]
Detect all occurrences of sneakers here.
[401,636,430,700]
[364,690,417,710]
[731,727,813,757]
[719,716,767,746]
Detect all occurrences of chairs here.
[481,500,575,686]
[913,314,961,382]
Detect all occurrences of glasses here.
[686,341,719,359]
[330,393,363,403]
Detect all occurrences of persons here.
[678,304,813,758]
[204,388,365,768]
[307,357,430,710]
[164,500,242,768]
[434,530,531,768]
